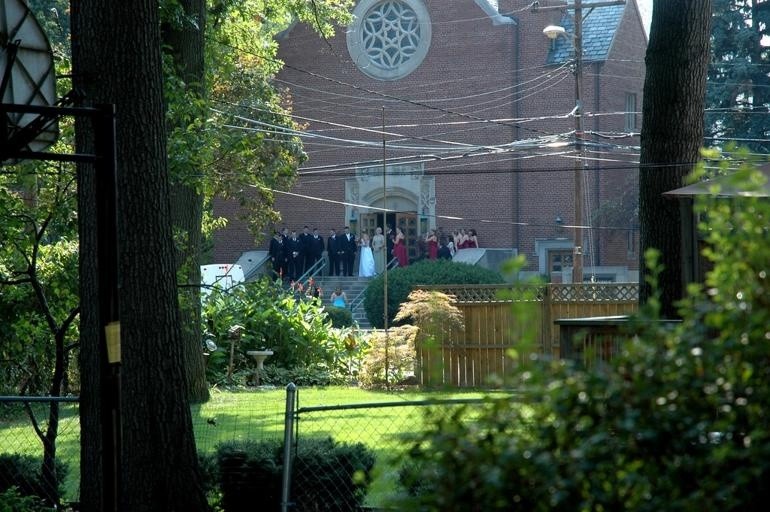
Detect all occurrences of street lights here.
[541,23,586,284]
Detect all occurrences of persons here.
[288,277,349,309]
[269,224,479,279]
